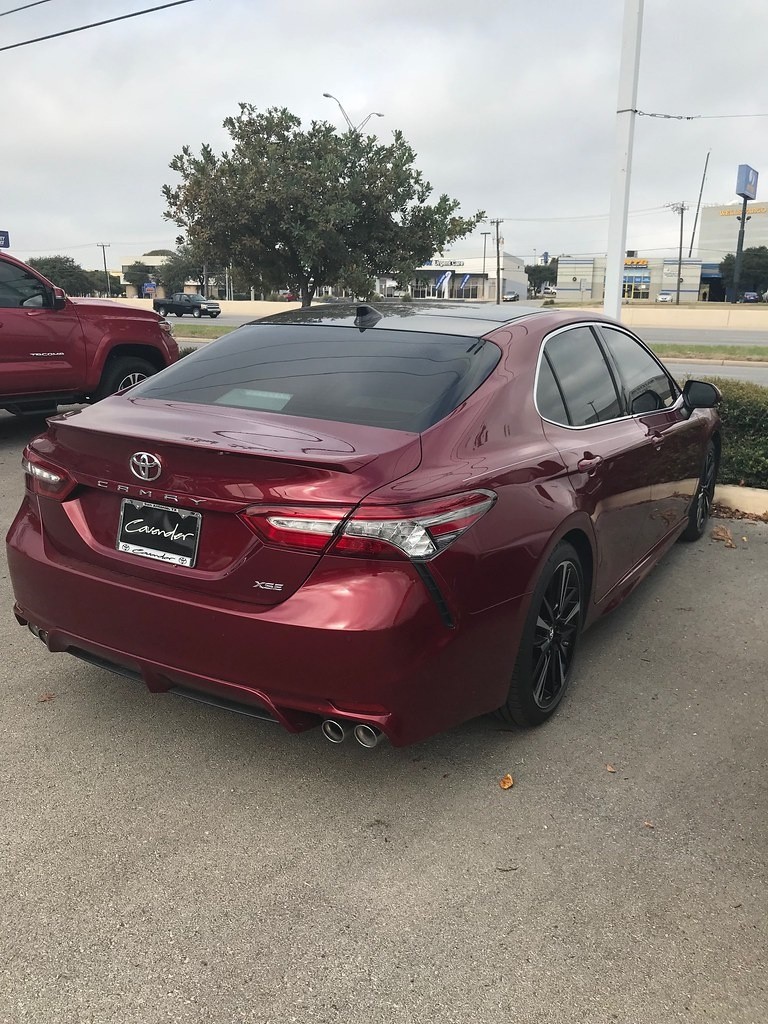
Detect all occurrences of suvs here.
[0,253,179,414]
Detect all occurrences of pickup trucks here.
[153,292,221,318]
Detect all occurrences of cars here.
[739,292,757,303]
[4,299,726,752]
[656,293,673,303]
[503,291,519,302]
[282,293,299,302]
[538,287,556,295]
